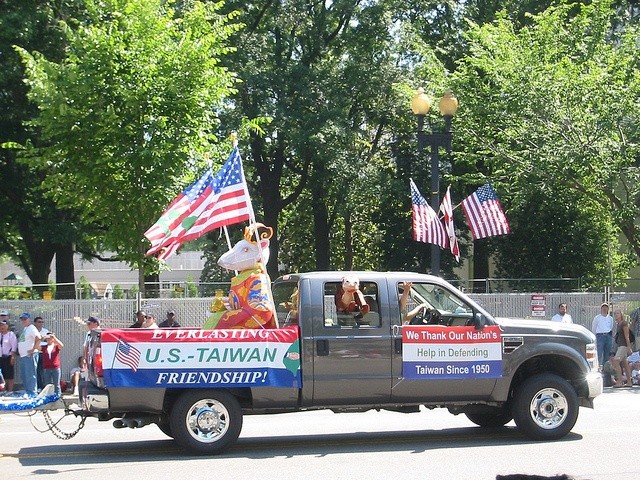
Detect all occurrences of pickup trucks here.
[34,272,603,456]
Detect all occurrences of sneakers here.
[614,383,624,387]
[623,382,632,387]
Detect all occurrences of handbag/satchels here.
[615,328,635,343]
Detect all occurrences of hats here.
[84,317,99,325]
[167,311,175,314]
[144,316,153,318]
[0,320,7,324]
[0,310,10,315]
[601,303,609,307]
[20,312,30,318]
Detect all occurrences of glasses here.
[38,321,44,323]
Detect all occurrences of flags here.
[146,148,253,264]
[439,182,460,263]
[143,167,219,263]
[409,179,451,251]
[461,183,511,238]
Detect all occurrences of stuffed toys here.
[338,277,370,309]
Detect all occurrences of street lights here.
[410,86,458,278]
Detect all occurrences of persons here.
[142,314,160,331]
[131,312,146,328]
[69,357,91,393]
[550,304,571,322]
[398,284,411,316]
[33,316,48,393]
[84,317,103,378]
[160,311,182,328]
[603,353,618,386]
[34,332,64,399]
[17,313,42,397]
[614,310,634,389]
[0,320,18,396]
[592,302,615,386]
[0,311,20,339]
